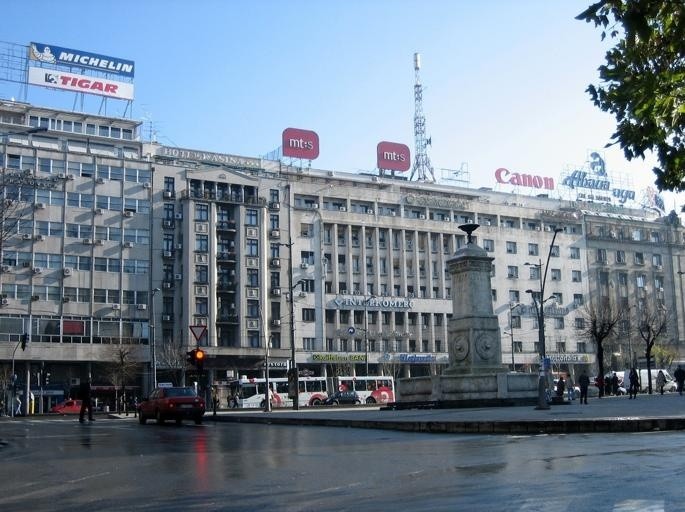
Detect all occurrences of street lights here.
[525,228,563,357]
[363,296,377,376]
[288,279,307,410]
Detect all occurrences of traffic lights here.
[43,371,50,386]
[22,332,30,351]
[186,350,204,368]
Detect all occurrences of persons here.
[231,392,240,408]
[627,368,640,400]
[78,380,97,423]
[578,369,590,405]
[14,394,23,415]
[227,395,232,408]
[598,376,605,399]
[557,376,565,396]
[604,376,612,396]
[673,364,685,396]
[264,387,274,413]
[657,370,666,395]
[611,372,619,396]
[565,374,577,401]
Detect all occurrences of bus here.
[229,376,396,408]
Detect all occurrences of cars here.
[326,390,361,405]
[138,383,205,425]
[553,372,599,398]
[51,399,83,414]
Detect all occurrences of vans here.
[604,369,677,398]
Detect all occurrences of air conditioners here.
[138,304,147,310]
[96,208,104,215]
[162,189,318,326]
[23,233,32,239]
[37,202,45,209]
[34,267,42,274]
[2,298,10,305]
[112,304,119,310]
[58,172,66,179]
[37,235,46,241]
[83,239,92,244]
[3,266,11,272]
[67,174,75,180]
[125,211,134,218]
[24,169,32,175]
[143,182,151,189]
[6,199,15,206]
[95,240,105,246]
[98,177,105,184]
[63,267,73,276]
[125,242,133,248]
[337,205,553,231]
